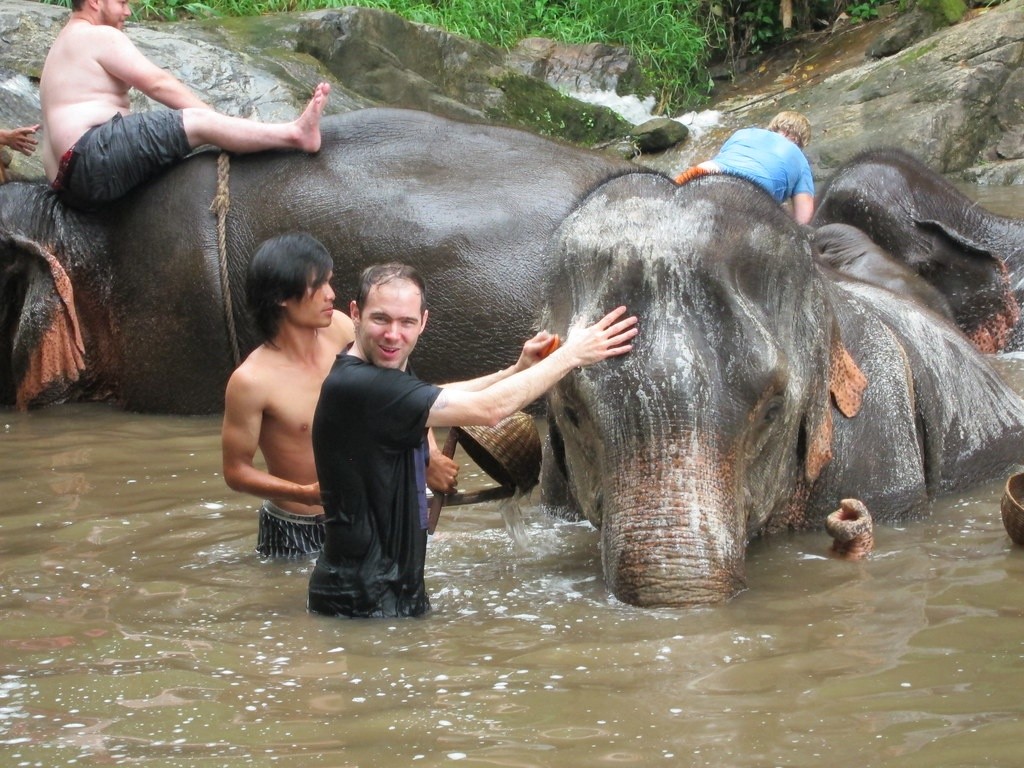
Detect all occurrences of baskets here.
[1000,472,1024,541]
[425,409,542,534]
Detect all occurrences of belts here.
[62,126,97,189]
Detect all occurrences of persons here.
[0,124,39,157]
[303,263,638,621]
[40,0,331,213]
[221,231,458,557]
[677,111,815,225]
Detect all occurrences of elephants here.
[803,146,1023,355]
[0,106,651,417]
[536,167,1024,609]
